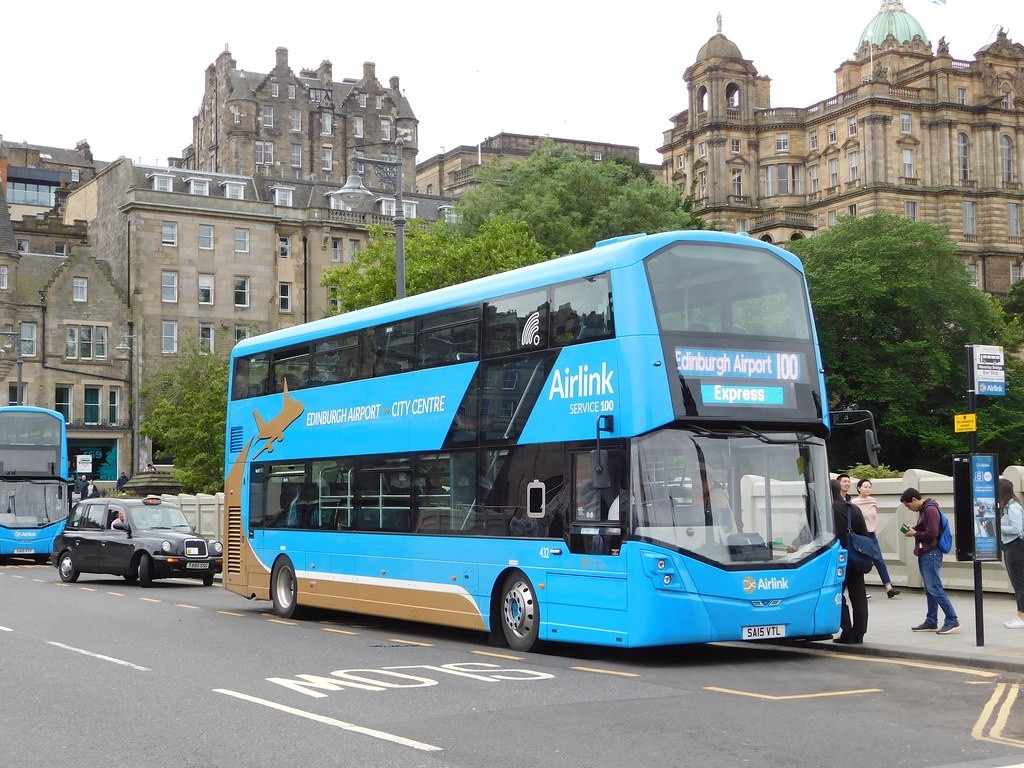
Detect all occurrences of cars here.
[51,497,223,588]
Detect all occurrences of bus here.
[223,230,881,652]
[0,406,69,564]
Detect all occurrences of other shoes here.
[936,621,960,634]
[1002,617,1019,627]
[853,628,863,644]
[833,629,856,644]
[1007,617,1024,629]
[866,593,872,599]
[912,621,938,630]
[887,589,900,598]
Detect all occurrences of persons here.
[74,475,89,494]
[508,507,549,537]
[117,472,129,493]
[851,479,901,599]
[111,511,124,529]
[976,504,986,517]
[900,487,960,634]
[144,464,151,471]
[997,480,1024,629]
[788,474,869,645]
[695,470,737,537]
[607,478,642,534]
[82,479,98,499]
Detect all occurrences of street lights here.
[116,335,139,473]
[334,137,407,300]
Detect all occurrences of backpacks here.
[923,502,953,554]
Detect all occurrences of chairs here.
[689,324,712,332]
[244,353,477,398]
[729,324,748,335]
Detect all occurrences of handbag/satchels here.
[847,529,876,574]
